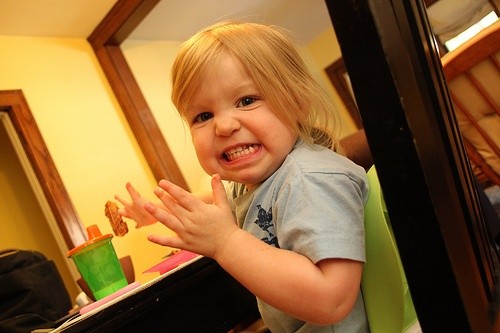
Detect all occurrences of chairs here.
[441,20,500,188]
[361,164,418,333]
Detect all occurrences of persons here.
[114,22,369,332]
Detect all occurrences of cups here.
[66,225,129,301]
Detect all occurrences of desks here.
[49,254,262,333]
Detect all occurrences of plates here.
[141,250,202,276]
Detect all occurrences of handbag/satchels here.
[0,249,73,333]
